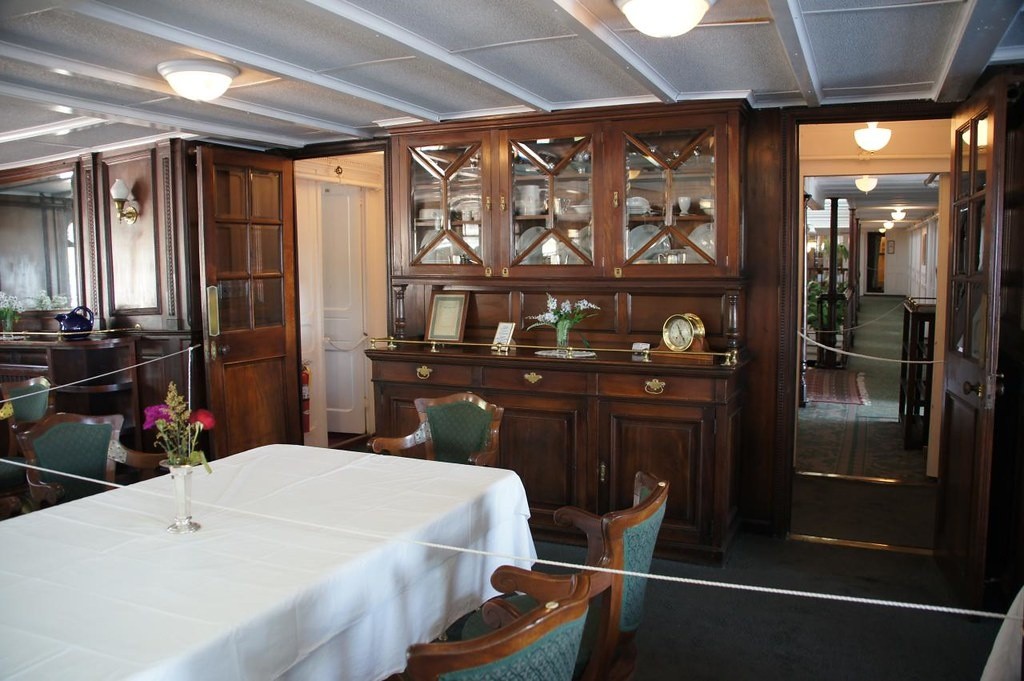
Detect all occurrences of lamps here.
[854,122,892,155]
[855,175,877,196]
[613,0,718,39]
[110,179,137,225]
[879,208,907,233]
[157,59,240,101]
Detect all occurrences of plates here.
[627,225,671,264]
[570,226,592,264]
[684,223,716,263]
[517,226,549,265]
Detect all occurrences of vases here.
[556,323,571,353]
[2,320,14,340]
[159,459,201,534]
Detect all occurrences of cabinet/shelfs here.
[0,334,143,449]
[364,101,759,569]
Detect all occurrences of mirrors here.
[0,160,80,319]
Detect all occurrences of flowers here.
[26,290,67,310]
[0,290,25,330]
[526,292,601,349]
[143,381,212,474]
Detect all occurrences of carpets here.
[805,367,872,405]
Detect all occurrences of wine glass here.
[678,196,691,216]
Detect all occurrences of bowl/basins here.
[572,204,592,213]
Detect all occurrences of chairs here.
[396,572,590,681]
[367,393,505,466]
[12,411,167,509]
[0,375,56,498]
[455,468,673,681]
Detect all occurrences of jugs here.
[54,306,95,339]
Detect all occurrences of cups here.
[518,185,548,215]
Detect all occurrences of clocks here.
[650,312,717,365]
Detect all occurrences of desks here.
[0,444,538,681]
